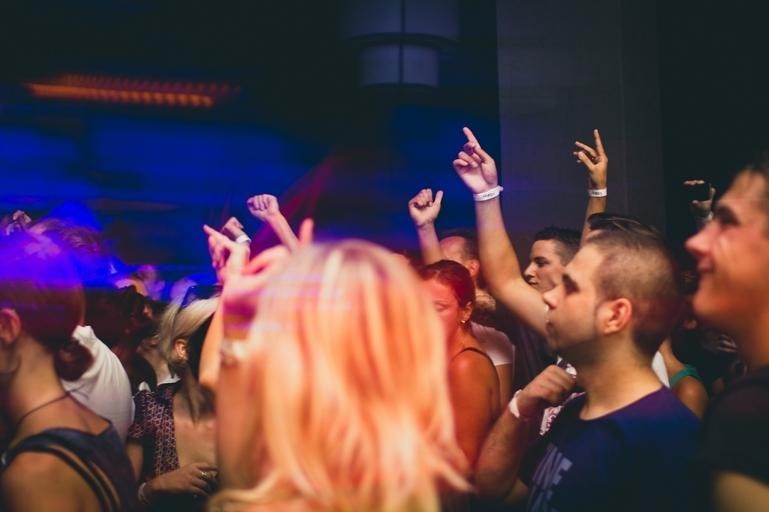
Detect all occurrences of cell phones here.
[690,182,712,202]
